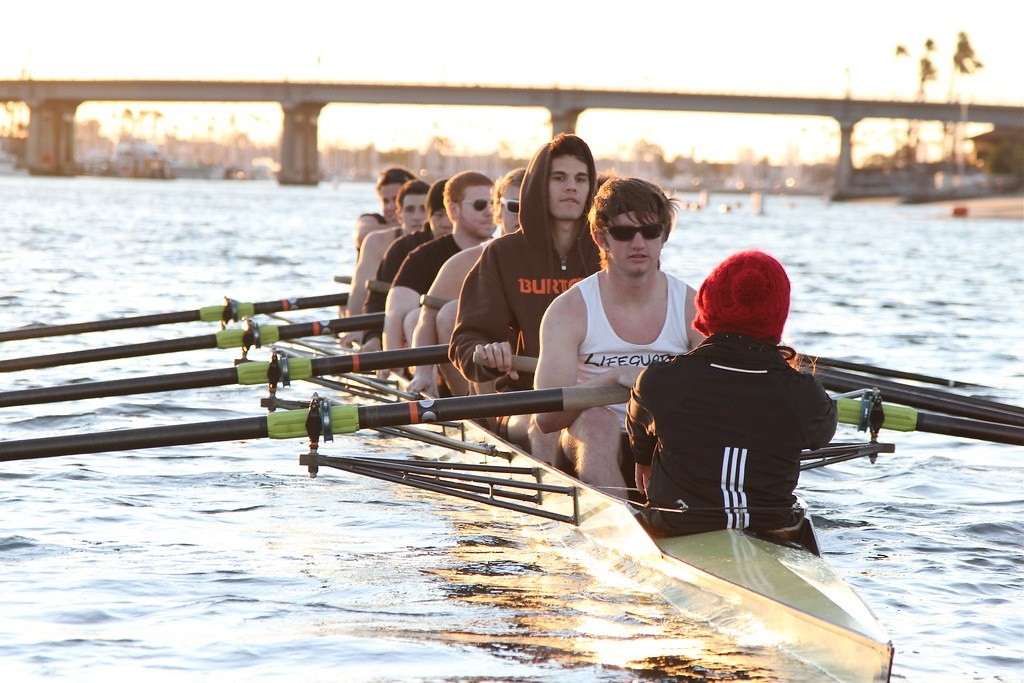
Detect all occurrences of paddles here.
[332,275,354,284]
[362,278,393,294]
[1,292,352,344]
[0,343,453,412]
[470,350,540,373]
[0,382,637,464]
[418,292,452,311]
[1,304,402,372]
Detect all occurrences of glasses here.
[604,224,665,241]
[500,197,520,215]
[458,199,494,212]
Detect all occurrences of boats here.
[326,320,895,683]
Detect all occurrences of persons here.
[624,250,837,543]
[533,176,705,498]
[342,168,527,429]
[448,132,605,465]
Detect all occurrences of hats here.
[690,251,791,345]
[426,179,446,217]
